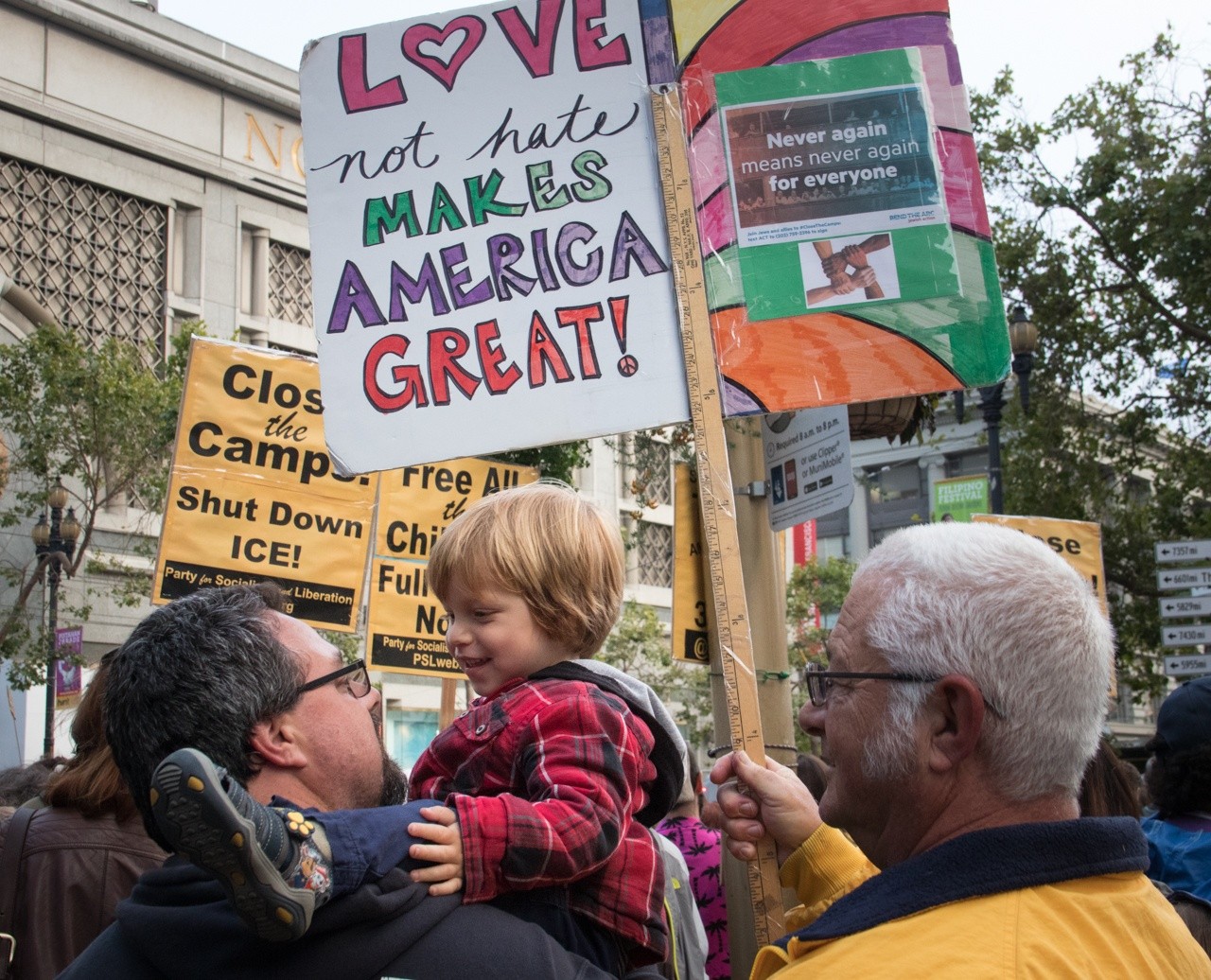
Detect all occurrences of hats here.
[1144,678,1211,749]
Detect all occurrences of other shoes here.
[150,748,333,944]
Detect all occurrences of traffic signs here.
[1155,538,1211,676]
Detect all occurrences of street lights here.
[951,302,1037,514]
[31,472,81,759]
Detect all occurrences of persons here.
[806,234,891,306]
[1,478,1211,980]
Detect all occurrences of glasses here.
[294,659,371,699]
[800,661,1007,724]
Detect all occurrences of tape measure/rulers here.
[651,89,786,951]
